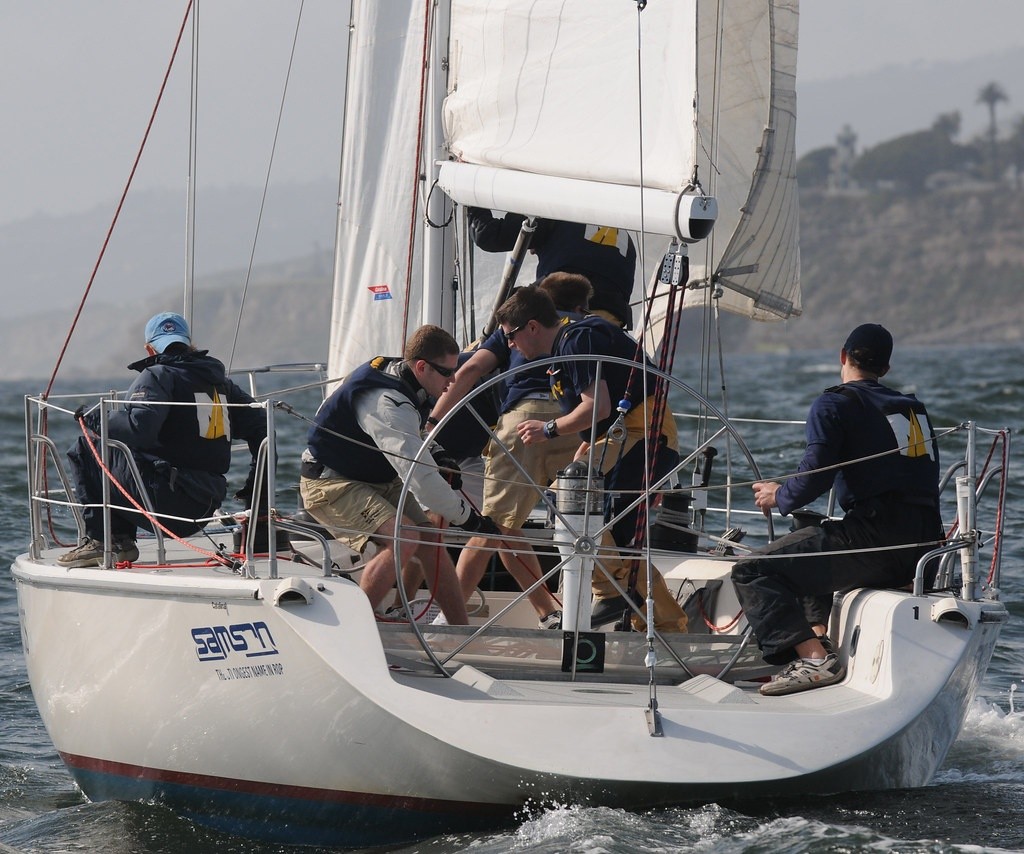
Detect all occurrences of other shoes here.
[423,611,449,643]
[538,610,562,654]
[592,586,644,627]
[632,642,681,664]
[385,606,414,620]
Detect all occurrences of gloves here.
[233,463,268,510]
[432,450,463,490]
[74,405,110,434]
[459,507,503,535]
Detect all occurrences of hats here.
[145,312,191,354]
[844,323,893,366]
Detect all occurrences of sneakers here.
[758,653,845,695]
[57,535,139,568]
[817,634,836,654]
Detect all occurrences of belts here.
[521,392,557,400]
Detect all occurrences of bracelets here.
[427,416,438,426]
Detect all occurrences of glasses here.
[415,355,459,377]
[504,315,541,340]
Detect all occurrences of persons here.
[421,207,635,436]
[426,270,595,630]
[496,285,690,630]
[297,324,502,625]
[56,313,279,568]
[729,321,947,696]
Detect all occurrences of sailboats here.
[8,0,1010,848]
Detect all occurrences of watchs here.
[543,419,560,439]
